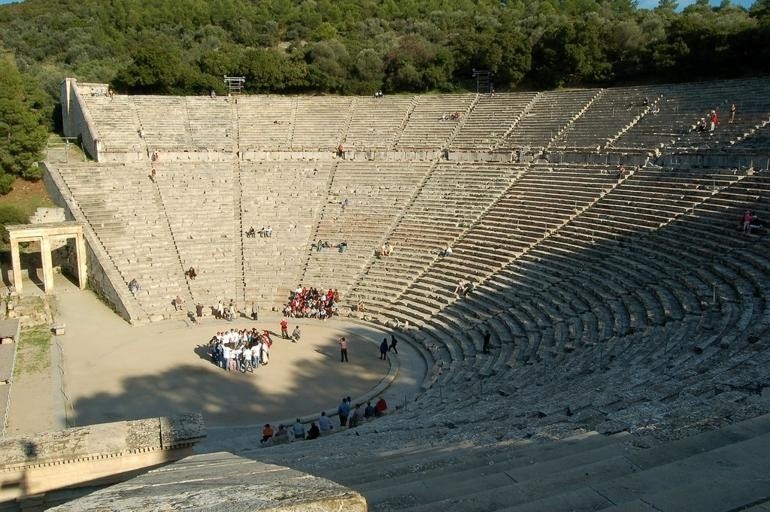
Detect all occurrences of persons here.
[261,224,266,238]
[260,396,388,443]
[175,296,183,311]
[380,243,386,255]
[695,110,719,134]
[251,300,259,320]
[195,302,204,320]
[291,325,301,342]
[282,283,340,319]
[249,225,255,237]
[463,281,475,296]
[742,210,752,231]
[388,335,398,354]
[280,319,289,339]
[730,103,736,121]
[339,337,349,362]
[208,327,273,374]
[445,246,453,258]
[189,267,197,280]
[265,225,272,237]
[385,244,392,256]
[317,239,322,252]
[340,240,347,253]
[378,338,389,360]
[750,211,761,234]
[440,110,460,122]
[213,297,236,322]
[618,165,625,178]
[453,277,465,296]
